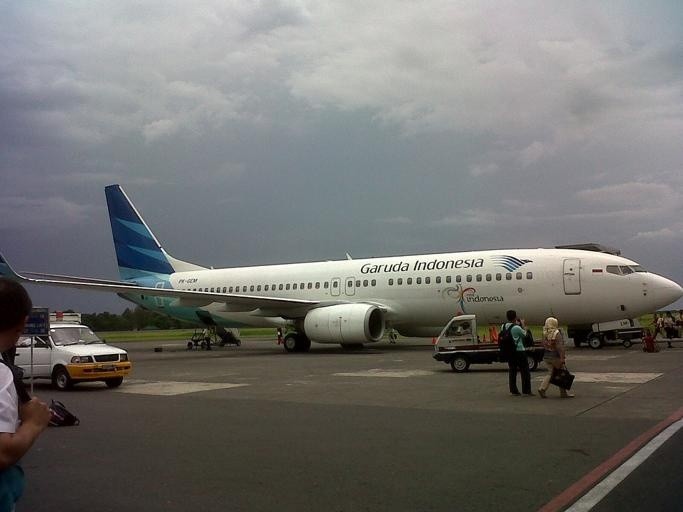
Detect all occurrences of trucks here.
[432,314,546,372]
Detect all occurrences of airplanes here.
[0,182,683,355]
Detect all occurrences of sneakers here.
[568,394,576,398]
[510,393,537,396]
[538,389,546,398]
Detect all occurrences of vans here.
[13,310,131,391]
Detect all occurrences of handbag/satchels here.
[47,400,80,426]
[549,366,575,390]
[0,463,24,511]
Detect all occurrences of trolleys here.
[640,327,661,353]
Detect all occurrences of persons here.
[537,315,576,399]
[450,322,472,336]
[499,310,535,396]
[0,276,53,510]
[647,308,682,349]
[385,328,396,345]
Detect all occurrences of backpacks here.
[498,323,520,362]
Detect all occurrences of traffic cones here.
[489,326,498,344]
